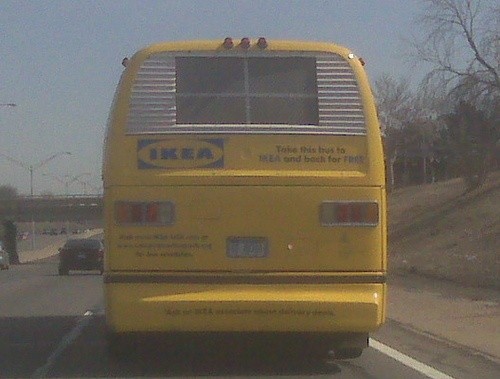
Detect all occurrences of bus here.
[100,36,390,361]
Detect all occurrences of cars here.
[0,240,10,269]
[56,238,103,276]
